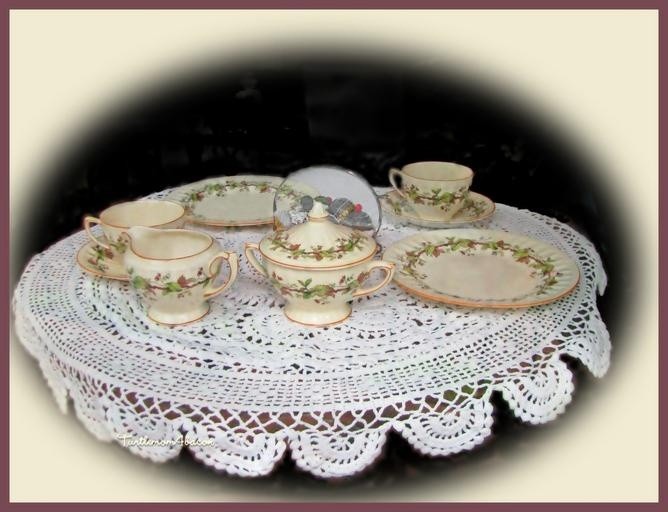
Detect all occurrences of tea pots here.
[121,221,238,327]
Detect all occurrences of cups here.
[390,161,475,222]
[82,198,187,252]
[244,199,396,328]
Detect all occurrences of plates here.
[375,186,496,229]
[377,225,582,310]
[73,227,139,281]
[270,164,383,245]
[163,171,283,227]
